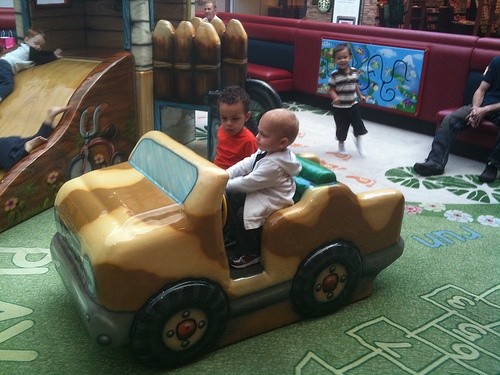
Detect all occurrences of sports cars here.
[49,129,405,370]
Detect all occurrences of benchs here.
[248,16,301,91]
[435,38,500,149]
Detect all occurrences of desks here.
[451,19,476,34]
[154,100,219,161]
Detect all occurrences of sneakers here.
[229,252,261,269]
[224,230,238,247]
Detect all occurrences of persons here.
[328,43,368,159]
[413,55,500,183]
[0,105,73,172]
[203,0,226,28]
[211,85,259,170]
[0,27,64,102]
[224,108,303,269]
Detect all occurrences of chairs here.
[293,152,337,205]
